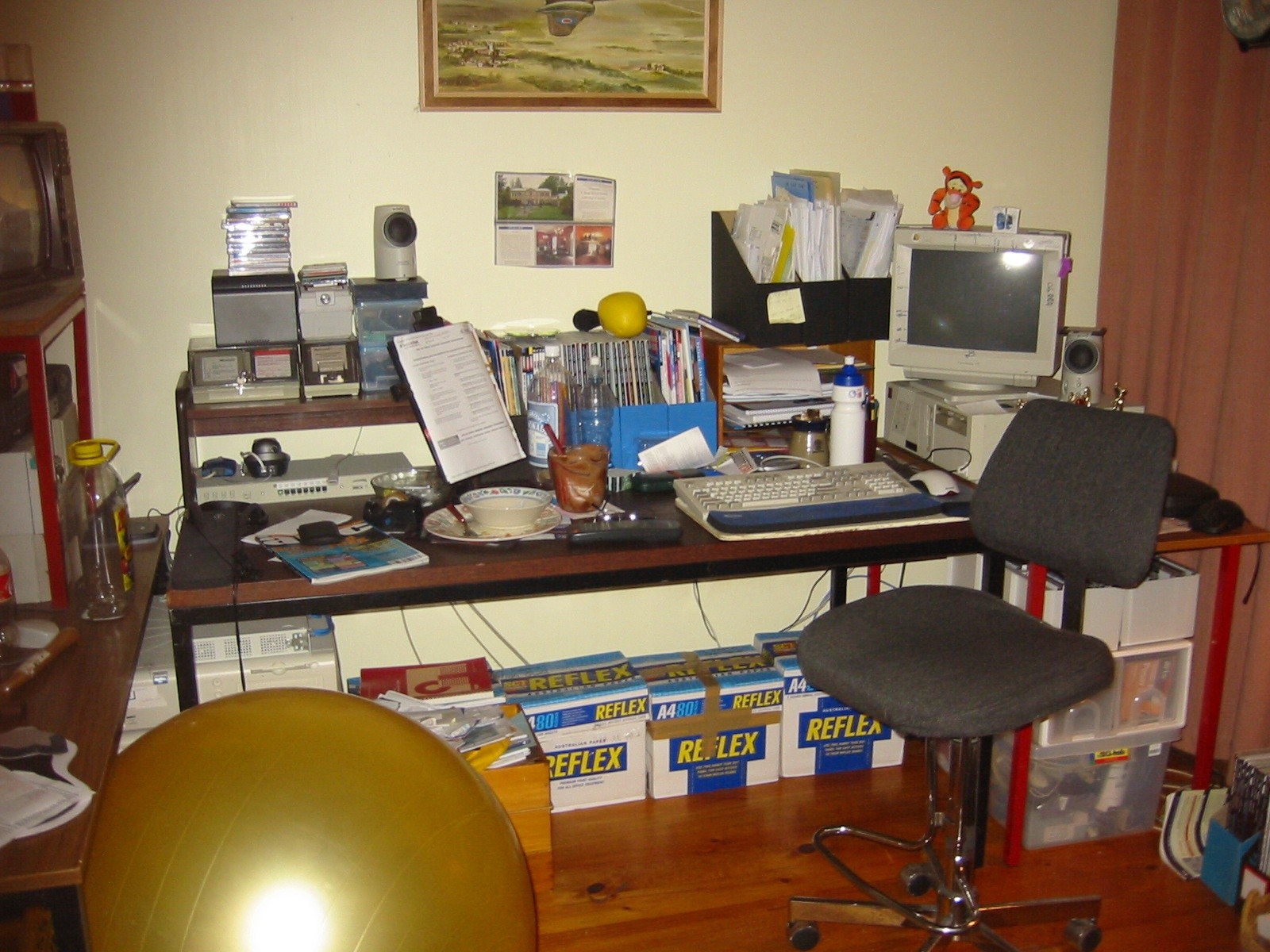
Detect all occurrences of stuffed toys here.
[928,166,983,231]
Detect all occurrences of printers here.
[116,593,344,756]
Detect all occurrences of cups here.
[787,414,829,470]
[548,444,610,512]
[865,419,879,463]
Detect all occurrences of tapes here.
[380,492,407,508]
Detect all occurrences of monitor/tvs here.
[888,225,1070,403]
[0,121,87,310]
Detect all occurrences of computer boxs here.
[884,382,1147,483]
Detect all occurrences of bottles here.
[577,357,619,456]
[527,344,580,488]
[0,550,21,667]
[829,356,866,466]
[61,438,136,620]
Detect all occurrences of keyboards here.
[673,462,921,522]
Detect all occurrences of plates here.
[422,504,562,542]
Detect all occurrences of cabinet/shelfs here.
[702,325,876,459]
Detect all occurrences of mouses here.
[1187,499,1245,534]
[909,470,960,497]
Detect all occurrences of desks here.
[0,512,198,952]
[0,288,126,612]
[164,415,1270,872]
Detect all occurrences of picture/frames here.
[417,0,723,114]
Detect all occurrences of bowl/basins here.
[459,486,553,531]
[371,467,452,508]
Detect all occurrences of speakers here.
[372,204,417,281]
[1061,332,1103,404]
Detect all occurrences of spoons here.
[443,501,477,537]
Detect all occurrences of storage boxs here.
[948,525,1200,653]
[188,336,301,405]
[295,282,354,338]
[1200,799,1270,918]
[997,640,1194,747]
[297,338,362,401]
[106,592,907,891]
[212,268,299,349]
[711,209,893,345]
[355,298,424,394]
[936,721,1182,848]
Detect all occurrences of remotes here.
[568,519,684,545]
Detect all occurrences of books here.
[722,345,867,453]
[388,309,745,484]
[1227,754,1270,877]
[1121,654,1177,720]
[360,657,531,770]
[255,518,429,586]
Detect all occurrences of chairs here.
[781,396,1179,952]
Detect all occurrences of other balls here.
[81,688,539,952]
